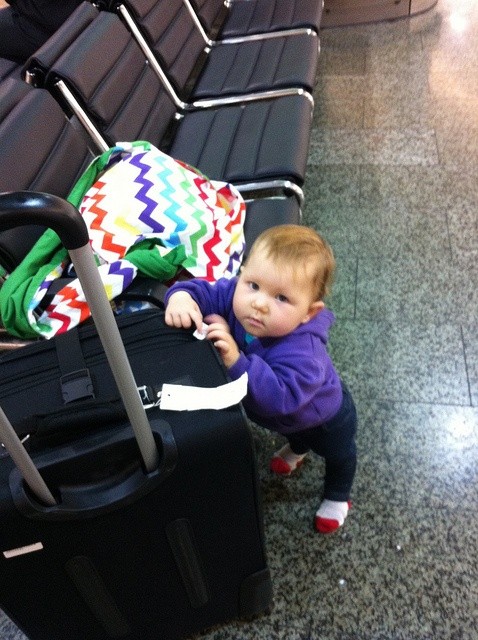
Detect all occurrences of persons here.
[164,224,357,533]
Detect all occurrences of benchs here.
[0,0,324,315]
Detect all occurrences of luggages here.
[1,191,273,638]
